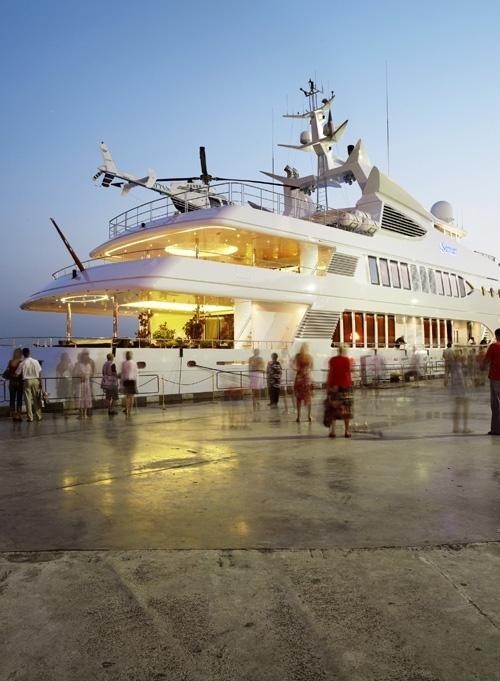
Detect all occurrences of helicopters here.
[79,140,307,220]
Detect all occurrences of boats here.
[0,60,500,410]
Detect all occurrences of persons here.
[6,347,27,422]
[55,352,74,419]
[321,343,356,439]
[71,349,94,418]
[480,329,499,436]
[266,353,286,410]
[248,348,266,413]
[363,336,499,409]
[74,354,97,421]
[289,343,316,424]
[118,352,139,417]
[443,343,474,434]
[100,354,123,416]
[13,348,46,422]
